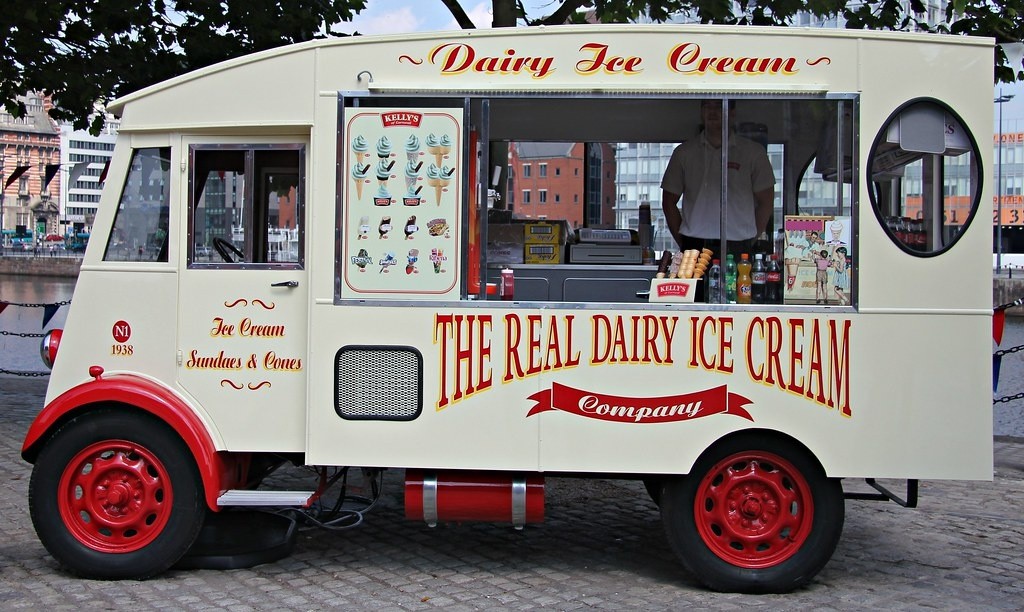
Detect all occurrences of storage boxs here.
[525,244,560,264]
[488,208,512,224]
[783,215,851,305]
[489,223,523,243]
[524,221,560,244]
[486,243,525,264]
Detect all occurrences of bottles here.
[726,254,737,304]
[643,247,655,266]
[767,255,771,266]
[709,260,720,304]
[775,229,784,263]
[499,267,514,301]
[766,256,780,304]
[750,254,766,304]
[737,254,751,304]
[885,215,927,250]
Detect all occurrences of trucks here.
[20,17,999,596]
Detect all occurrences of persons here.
[660,99,776,266]
[31,243,58,257]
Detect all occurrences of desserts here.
[404,184,420,198]
[373,184,391,198]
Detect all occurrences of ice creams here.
[406,134,421,161]
[405,159,419,190]
[352,135,368,163]
[376,136,392,161]
[375,157,390,188]
[352,162,367,200]
[427,132,451,167]
[427,163,452,206]
